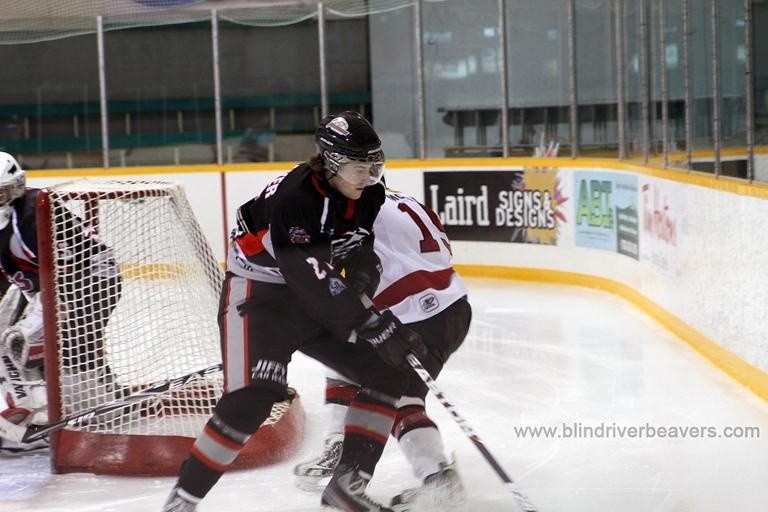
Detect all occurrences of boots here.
[290,434,345,477]
[392,450,461,506]
[164,458,203,512]
[320,441,392,512]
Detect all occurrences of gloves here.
[354,308,428,372]
[351,249,383,300]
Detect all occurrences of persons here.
[0,151,132,461]
[294,172,472,510]
[159,110,428,512]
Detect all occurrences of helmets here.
[315,111,386,187]
[0,152,28,232]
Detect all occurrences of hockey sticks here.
[0,364,225,444]
